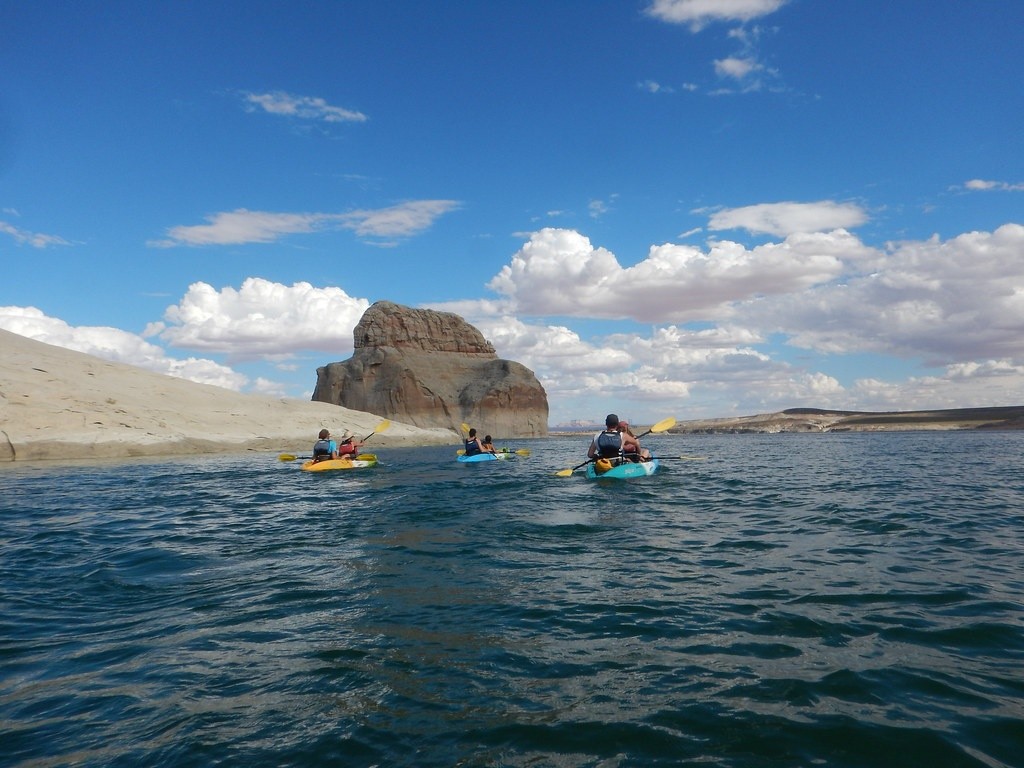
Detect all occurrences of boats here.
[457,447,511,462]
[585,446,659,480]
[300,453,377,470]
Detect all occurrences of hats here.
[606,414,619,426]
[319,428,329,439]
[341,430,354,441]
[619,421,628,427]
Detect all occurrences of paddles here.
[456,449,531,457]
[355,419,390,448]
[279,454,313,462]
[649,455,709,461]
[459,422,500,461]
[557,416,676,477]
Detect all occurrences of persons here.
[339,431,363,457]
[464,428,492,456]
[312,429,341,459]
[588,414,651,464]
[482,435,496,453]
